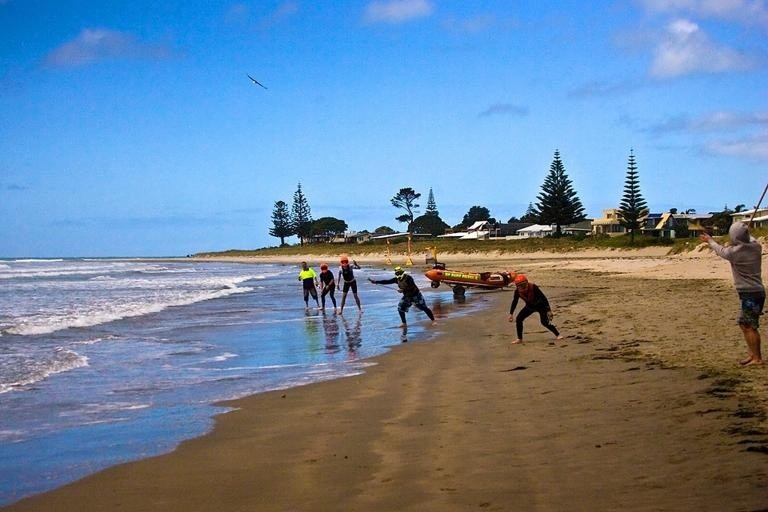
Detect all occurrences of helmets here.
[394,266,404,281]
[340,257,348,267]
[320,263,328,273]
[514,273,528,294]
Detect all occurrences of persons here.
[402,327,407,342]
[508,274,563,344]
[341,312,362,359]
[323,310,340,353]
[305,310,322,353]
[371,266,437,327]
[337,257,362,314]
[318,264,337,313]
[298,262,321,308]
[699,222,766,367]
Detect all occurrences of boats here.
[425,262,517,295]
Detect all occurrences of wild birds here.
[247,75,268,90]
